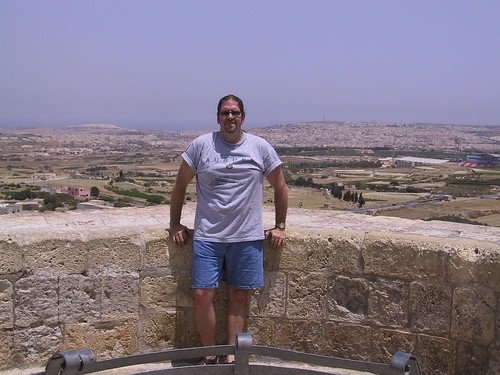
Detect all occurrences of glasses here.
[217,110,243,116]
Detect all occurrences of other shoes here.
[199,356,235,364]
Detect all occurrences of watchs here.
[275,222,286,230]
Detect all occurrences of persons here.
[170,95,288,365]
